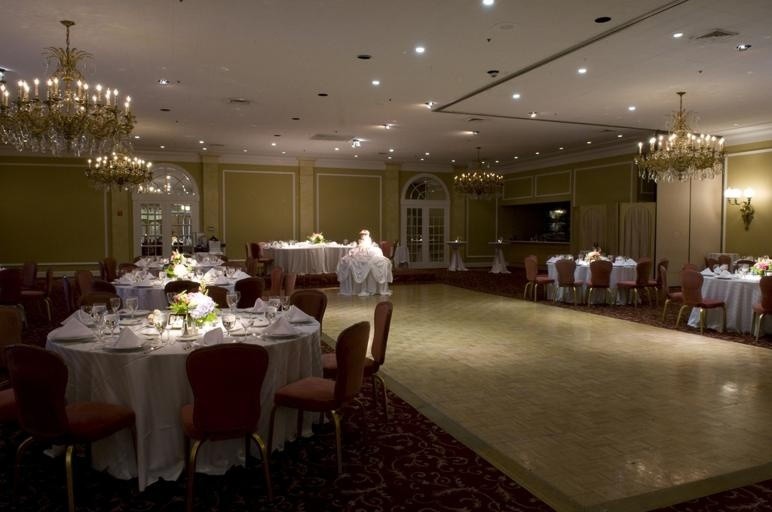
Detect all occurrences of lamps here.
[724,186,754,230]
[82,140,153,195]
[633,91,728,185]
[0,20,137,159]
[448,146,505,200]
[145,180,162,196]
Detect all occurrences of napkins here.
[55,318,96,338]
[252,298,272,312]
[262,318,301,335]
[61,309,94,324]
[276,305,314,322]
[114,326,144,348]
[201,328,224,345]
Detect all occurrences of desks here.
[244,240,395,297]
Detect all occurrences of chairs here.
[182,341,272,502]
[289,289,328,322]
[322,302,393,424]
[3,344,140,512]
[268,320,373,474]
[114,254,264,310]
[0,258,139,427]
[523,255,772,344]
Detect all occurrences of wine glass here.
[196,272,203,282]
[229,268,235,281]
[159,272,167,288]
[80,292,291,342]
[742,263,749,279]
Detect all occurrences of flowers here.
[173,292,217,320]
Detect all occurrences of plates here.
[290,319,314,325]
[269,329,304,339]
[103,344,144,352]
[110,282,154,288]
[53,321,93,342]
[700,274,731,279]
